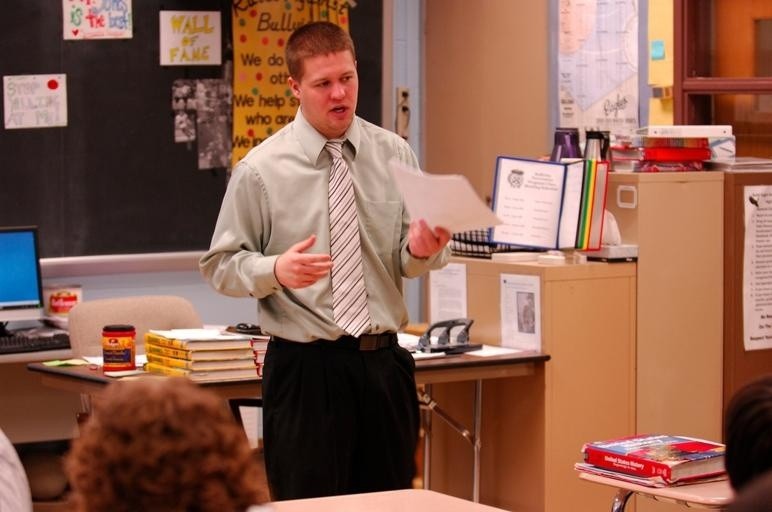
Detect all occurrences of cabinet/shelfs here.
[422,251,638,509]
[607,172,724,446]
[724,170,771,394]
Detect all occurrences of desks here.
[270,488,513,512]
[25,342,551,503]
[577,469,734,512]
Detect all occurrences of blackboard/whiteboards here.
[0,0,393,279]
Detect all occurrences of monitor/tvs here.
[0,224,44,309]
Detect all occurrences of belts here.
[270,331,400,353]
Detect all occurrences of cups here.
[41,285,82,316]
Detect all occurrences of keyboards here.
[0,333,72,354]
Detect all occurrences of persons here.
[62,375,272,512]
[722,375,772,512]
[198,22,452,501]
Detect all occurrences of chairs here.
[68,296,205,358]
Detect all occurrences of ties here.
[322,140,373,338]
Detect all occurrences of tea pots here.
[551,128,583,163]
[584,127,614,172]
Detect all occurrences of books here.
[143,327,270,381]
[573,432,727,489]
[609,123,737,172]
